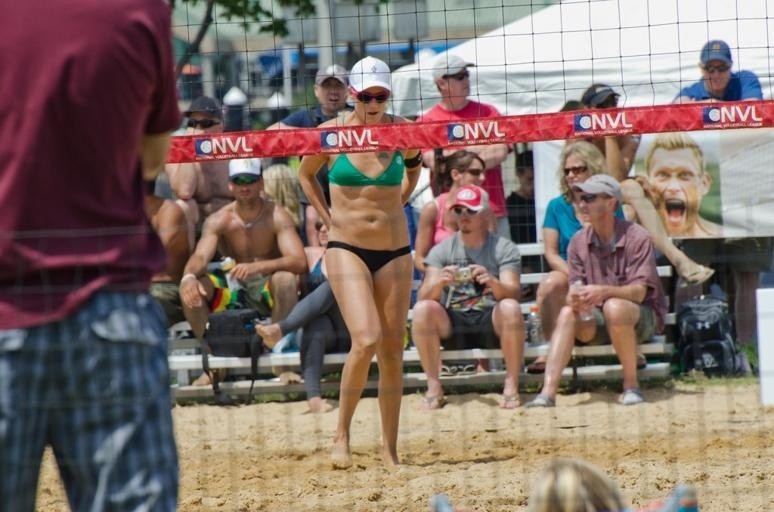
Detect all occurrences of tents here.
[346,0,774,243]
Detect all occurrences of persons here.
[558,84,716,287]
[296,55,422,472]
[2,0,182,510]
[529,460,624,512]
[403,51,514,307]
[505,149,541,303]
[671,41,764,105]
[523,173,667,408]
[646,133,725,237]
[144,63,355,413]
[527,140,647,371]
[412,183,525,410]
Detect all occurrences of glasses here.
[445,71,468,81]
[349,88,390,103]
[315,222,323,230]
[563,166,587,175]
[232,175,259,185]
[703,65,729,73]
[187,119,219,129]
[580,194,596,202]
[451,208,481,216]
[597,97,618,108]
[467,168,480,177]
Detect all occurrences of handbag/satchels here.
[201,308,261,358]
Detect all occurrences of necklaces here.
[233,197,264,230]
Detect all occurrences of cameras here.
[453,267,472,282]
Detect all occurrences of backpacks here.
[675,284,744,379]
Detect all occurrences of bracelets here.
[181,273,196,282]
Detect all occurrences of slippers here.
[502,380,521,408]
[684,264,715,286]
[525,394,555,407]
[458,362,476,375]
[527,360,545,373]
[439,361,457,376]
[421,395,447,410]
[621,388,642,405]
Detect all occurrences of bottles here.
[219,254,237,275]
[527,305,545,348]
[572,280,595,322]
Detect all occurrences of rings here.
[480,275,483,278]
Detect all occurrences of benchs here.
[167,243,677,402]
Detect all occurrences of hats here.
[228,157,262,178]
[349,56,390,93]
[581,84,621,107]
[183,96,222,118]
[448,183,490,211]
[571,174,622,202]
[314,64,348,90]
[432,54,474,82]
[701,41,731,65]
